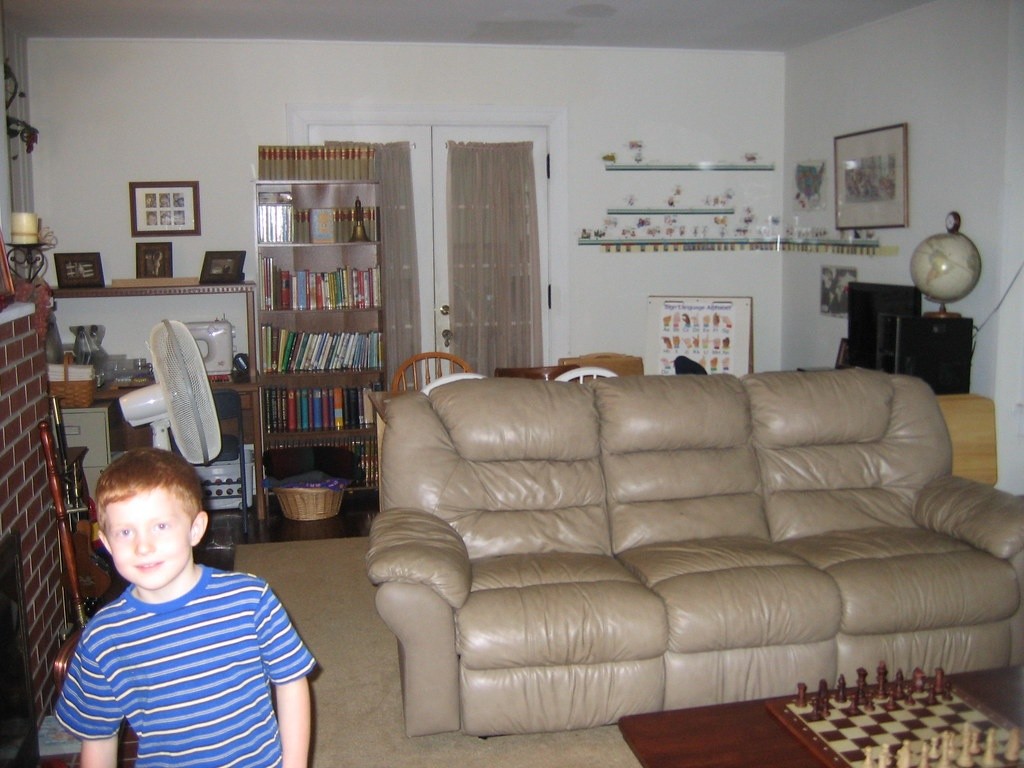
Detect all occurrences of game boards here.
[764,675,1024,768]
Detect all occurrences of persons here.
[146,192,185,225]
[54,445,317,768]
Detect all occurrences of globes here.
[909,233,982,319]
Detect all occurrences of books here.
[295,205,375,242]
[258,205,294,243]
[262,385,345,431]
[259,253,317,311]
[260,324,326,371]
[342,382,382,425]
[258,145,375,181]
[319,331,383,369]
[331,436,378,482]
[263,434,330,484]
[317,266,381,310]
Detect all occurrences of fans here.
[118,319,222,465]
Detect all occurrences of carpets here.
[233,531,644,768]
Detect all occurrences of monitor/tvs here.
[847,281,921,371]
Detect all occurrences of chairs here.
[673,354,710,375]
[169,388,248,535]
[390,351,472,393]
[558,353,643,383]
[554,365,618,382]
[495,364,581,382]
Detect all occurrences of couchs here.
[360,367,1024,745]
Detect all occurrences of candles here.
[10,212,38,244]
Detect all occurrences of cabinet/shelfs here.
[573,161,879,254]
[253,176,385,543]
[41,283,260,546]
[50,403,113,502]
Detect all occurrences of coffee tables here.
[619,664,1024,768]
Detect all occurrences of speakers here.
[911,318,973,395]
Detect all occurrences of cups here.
[133,358,153,377]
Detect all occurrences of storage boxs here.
[44,361,96,410]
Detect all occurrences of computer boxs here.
[877,311,913,375]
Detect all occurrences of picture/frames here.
[832,122,910,232]
[0,227,15,300]
[126,179,203,238]
[53,252,105,289]
[198,250,248,285]
[134,242,174,278]
